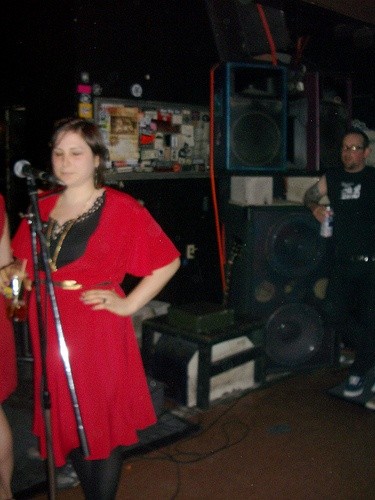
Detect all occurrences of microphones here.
[13,159,65,185]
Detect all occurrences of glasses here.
[342,144,365,151]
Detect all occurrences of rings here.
[103,298,107,303]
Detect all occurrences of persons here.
[6,115,180,500]
[303,129,375,406]
[0,194,27,500]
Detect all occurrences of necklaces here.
[45,189,95,271]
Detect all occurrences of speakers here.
[213,62,290,173]
[217,202,338,382]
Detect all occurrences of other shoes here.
[364,383,375,411]
[343,374,366,398]
